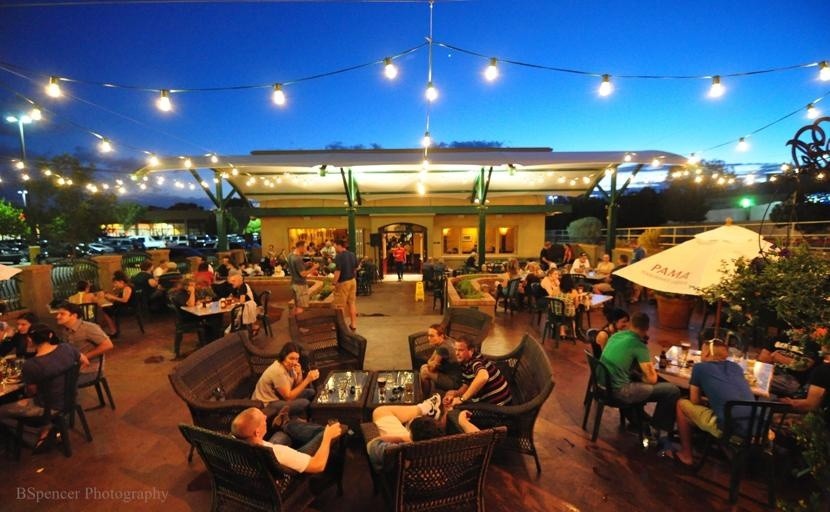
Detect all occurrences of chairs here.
[2,250,829,510]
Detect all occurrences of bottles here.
[659,350,666,369]
[2,356,25,384]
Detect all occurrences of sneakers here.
[626,413,672,445]
[270,404,292,430]
[424,390,442,422]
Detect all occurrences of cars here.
[0,239,25,266]
[15,233,262,257]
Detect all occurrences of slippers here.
[662,444,696,469]
[30,427,55,456]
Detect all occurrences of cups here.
[734,352,743,366]
[199,294,246,311]
[310,368,415,404]
[747,351,758,372]
[677,342,690,368]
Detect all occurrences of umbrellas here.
[612,214,785,338]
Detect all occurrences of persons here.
[419,323,463,401]
[12,323,90,450]
[52,297,112,454]
[592,306,829,469]
[249,340,321,418]
[439,336,514,433]
[230,403,344,479]
[0,313,58,357]
[363,391,482,490]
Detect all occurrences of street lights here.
[4,111,35,234]
[16,188,29,207]
